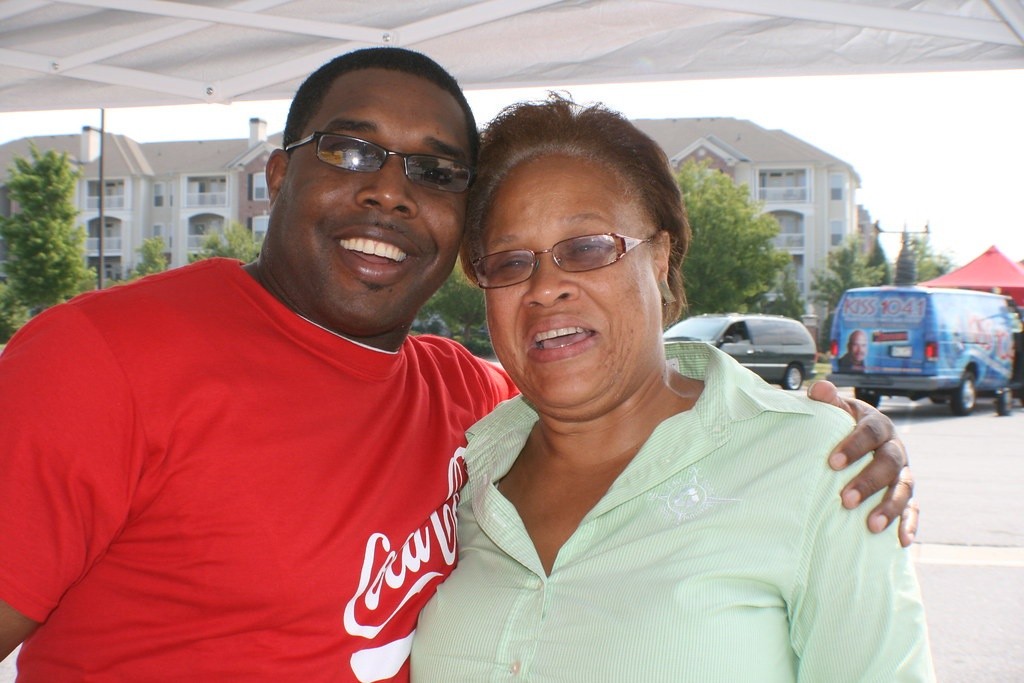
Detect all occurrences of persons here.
[0,48,920,683]
[838,329,867,373]
[408,101,935,683]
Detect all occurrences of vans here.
[825,286,1024,416]
[663,312,817,390]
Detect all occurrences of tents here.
[918,244,1024,306]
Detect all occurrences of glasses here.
[471,225,661,289]
[282,130,473,192]
